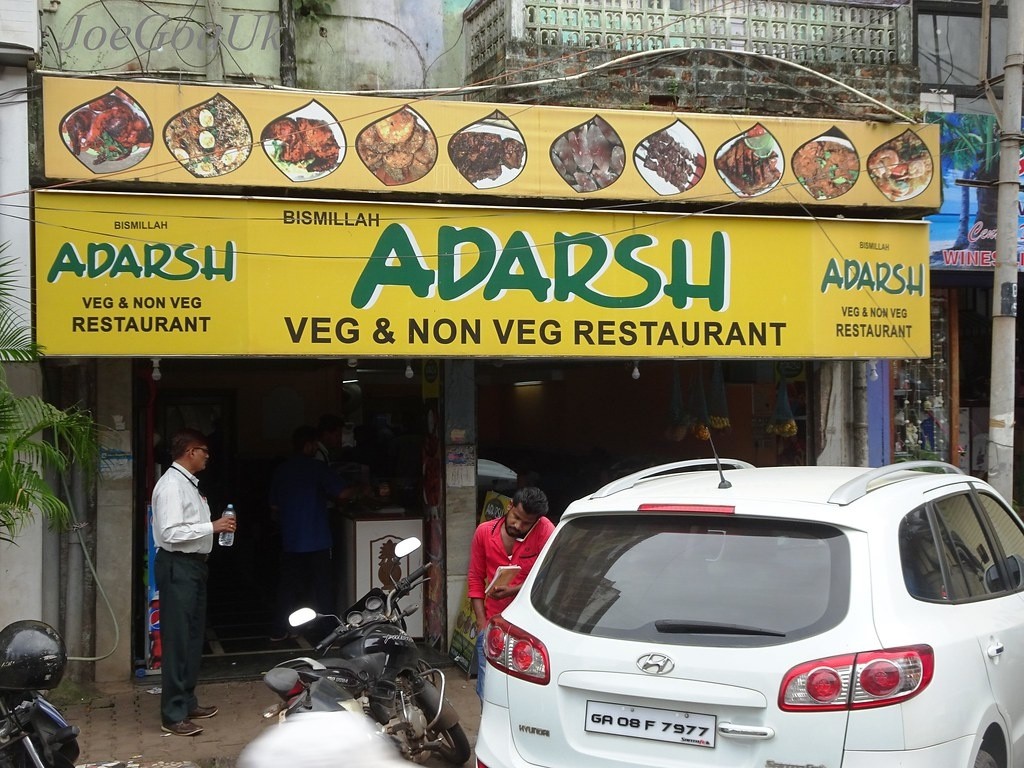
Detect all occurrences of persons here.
[468,485,556,706]
[151,428,236,736]
[270,415,352,647]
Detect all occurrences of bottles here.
[218,503,236,546]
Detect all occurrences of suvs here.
[471,456,1024,768]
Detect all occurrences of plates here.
[791,125,861,201]
[58,86,154,174]
[354,104,439,187]
[259,97,347,183]
[713,122,786,199]
[633,118,707,196]
[162,92,253,179]
[866,127,934,202]
[447,109,528,190]
[549,114,626,194]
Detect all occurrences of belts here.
[175,550,209,562]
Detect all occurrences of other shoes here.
[269,631,299,642]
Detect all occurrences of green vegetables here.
[96,132,126,159]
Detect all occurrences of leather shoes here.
[161,720,203,735]
[185,706,218,718]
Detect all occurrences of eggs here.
[199,131,215,148]
[199,109,214,127]
[174,148,189,164]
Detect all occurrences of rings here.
[230,525,234,528]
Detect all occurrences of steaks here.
[717,138,779,194]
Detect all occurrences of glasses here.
[185,447,210,455]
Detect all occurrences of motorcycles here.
[258,535,472,767]
